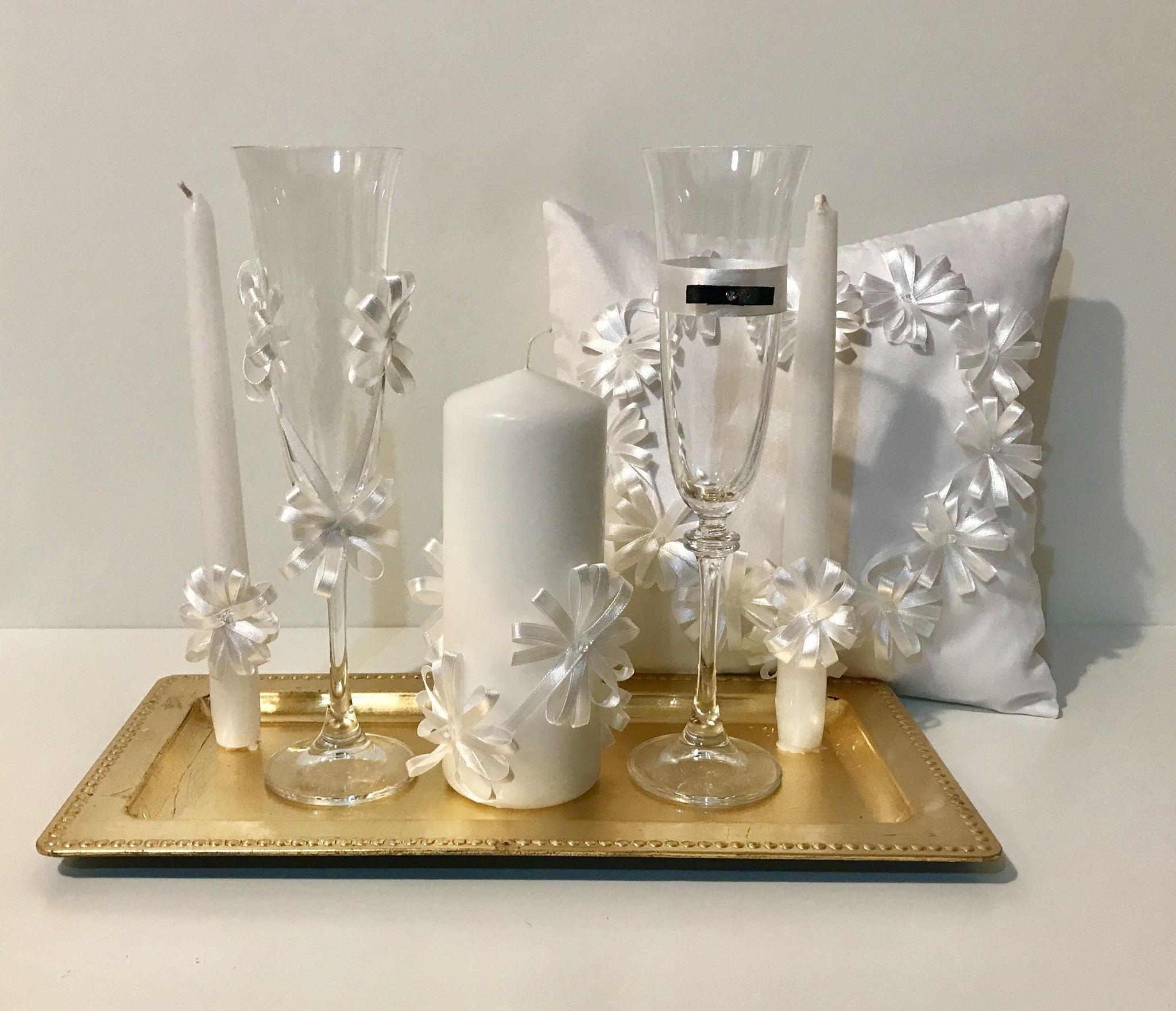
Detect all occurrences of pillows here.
[540,190,1071,721]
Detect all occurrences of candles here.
[176,180,264,752]
[439,330,612,811]
[772,193,840,754]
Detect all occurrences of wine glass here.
[233,145,416,806]
[626,144,814,808]
